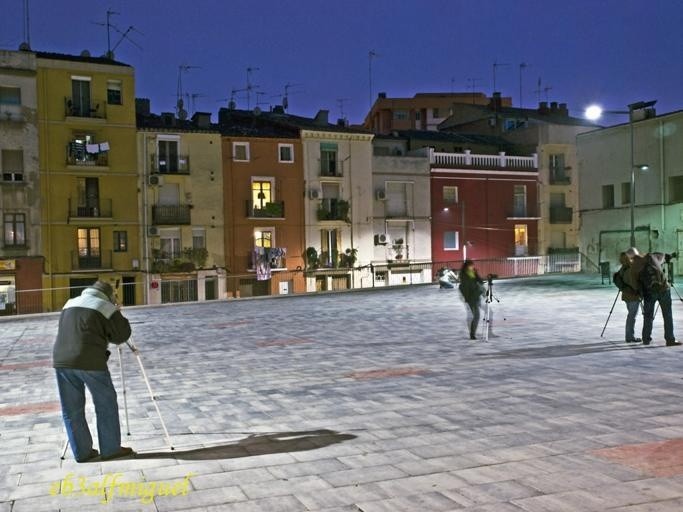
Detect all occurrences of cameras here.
[487,274,498,280]
[665,253,676,260]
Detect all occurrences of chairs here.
[67,99,81,116]
[88,104,100,118]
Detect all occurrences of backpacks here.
[612,267,628,293]
[637,255,667,297]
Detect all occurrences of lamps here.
[257,189,265,199]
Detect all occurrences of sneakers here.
[666,340,682,346]
[101,446,133,460]
[76,449,99,462]
[625,337,651,345]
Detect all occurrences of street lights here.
[584,105,650,247]
[443,204,467,265]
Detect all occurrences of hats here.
[87,280,113,302]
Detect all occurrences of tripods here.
[600,261,683,337]
[481,280,512,343]
[61,338,175,461]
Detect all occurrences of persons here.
[52,281,134,464]
[613,250,643,344]
[625,247,681,347]
[459,258,498,342]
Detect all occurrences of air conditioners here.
[149,175,163,186]
[376,190,389,200]
[378,233,391,243]
[147,226,160,236]
[310,189,323,200]
